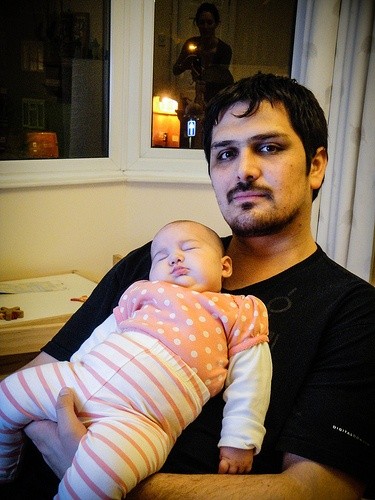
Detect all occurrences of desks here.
[0,273,99,356]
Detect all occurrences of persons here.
[0,220,272,500]
[173,2,234,105]
[0,71,375,500]
